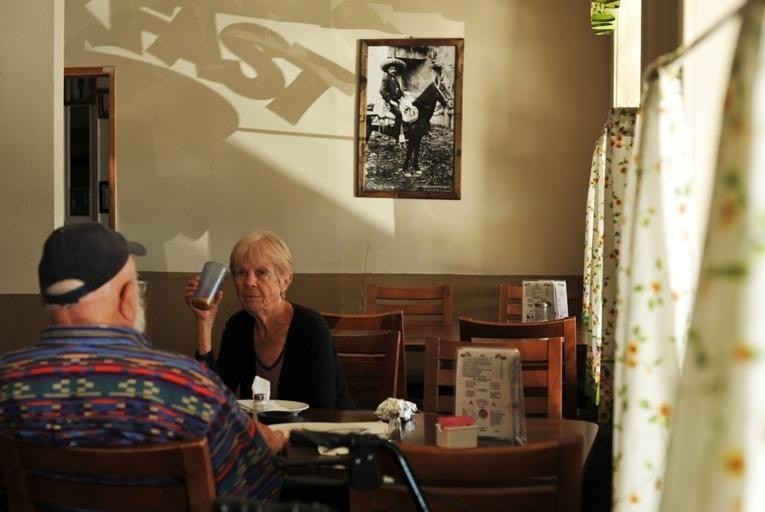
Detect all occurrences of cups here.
[191,261,229,311]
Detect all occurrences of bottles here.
[526,300,552,322]
[388,411,402,443]
[254,394,264,414]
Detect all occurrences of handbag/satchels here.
[400,101,419,122]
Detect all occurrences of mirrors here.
[64,65,116,233]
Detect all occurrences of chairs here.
[421,336,568,422]
[1,432,217,512]
[323,326,405,406]
[495,284,564,322]
[362,283,456,327]
[452,312,580,406]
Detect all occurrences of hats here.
[381,58,407,73]
[39,219,147,304]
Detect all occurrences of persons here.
[380,65,407,151]
[184,231,354,409]
[0,221,295,512]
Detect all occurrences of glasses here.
[117,279,148,297]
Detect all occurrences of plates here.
[236,399,310,416]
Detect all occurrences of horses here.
[366,74,454,177]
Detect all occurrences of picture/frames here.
[65,77,97,105]
[97,88,109,120]
[356,37,466,202]
[99,181,109,214]
